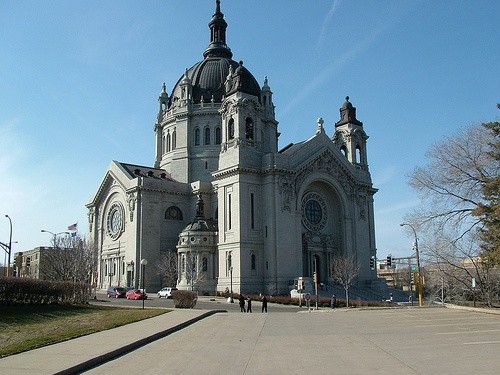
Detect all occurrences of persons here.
[261,296,267,312]
[239,298,246,312]
[331,294,336,309]
[246,297,252,313]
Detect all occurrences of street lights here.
[41,230,69,255]
[230,267,234,303]
[0,215,18,277]
[140,258,148,310]
[3,242,18,275]
[400,224,423,307]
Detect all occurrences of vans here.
[157,287,178,299]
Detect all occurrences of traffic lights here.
[410,273,415,284]
[14,255,23,268]
[22,257,30,266]
[387,255,392,266]
[294,279,305,291]
[313,273,317,283]
[370,256,375,270]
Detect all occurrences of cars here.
[107,287,128,298]
[126,289,147,300]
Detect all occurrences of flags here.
[68,224,77,230]
[71,232,76,237]
